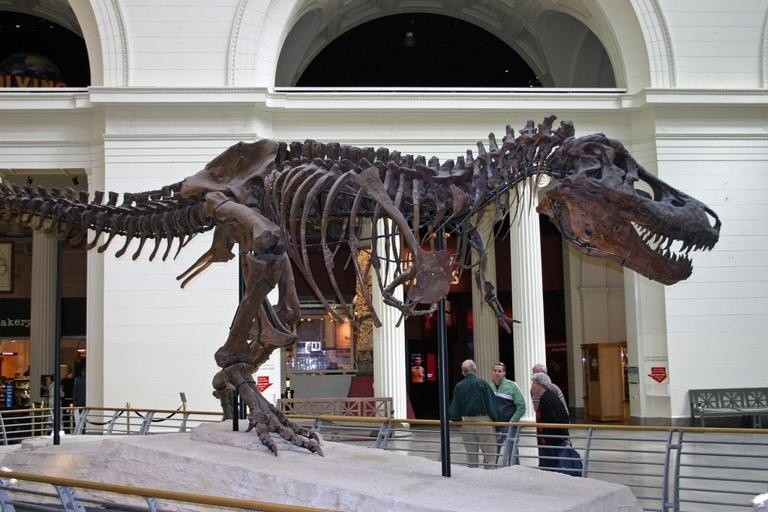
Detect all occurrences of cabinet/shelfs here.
[581,343,626,424]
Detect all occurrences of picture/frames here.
[0,241,15,293]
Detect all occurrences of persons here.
[448,358,526,470]
[530,363,573,472]
[45,364,86,430]
[411,356,426,383]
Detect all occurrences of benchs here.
[276,398,395,442]
[687,387,768,428]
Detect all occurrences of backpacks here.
[554,440,583,477]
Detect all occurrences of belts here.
[462,412,490,416]
